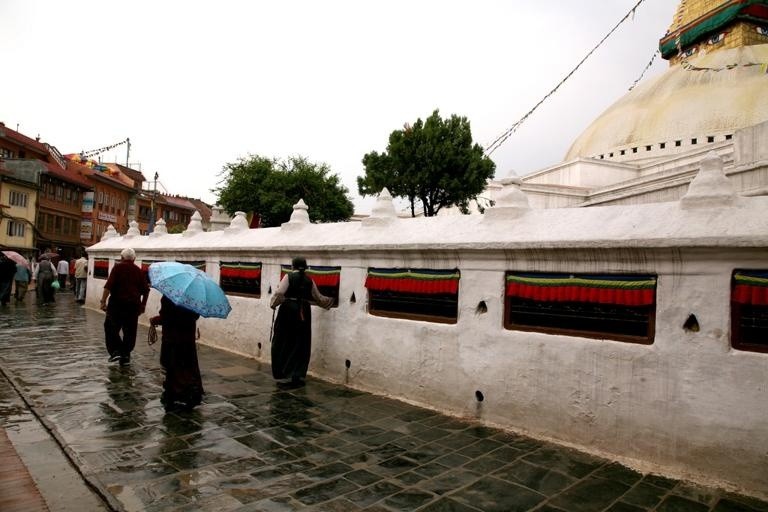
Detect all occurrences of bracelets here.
[100,300,106,303]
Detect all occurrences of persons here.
[0,251,88,307]
[269,256,335,389]
[150,294,205,410]
[99,248,150,366]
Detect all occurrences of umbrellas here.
[148,262,233,319]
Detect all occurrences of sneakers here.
[119,355,130,366]
[108,351,121,363]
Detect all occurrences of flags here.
[156,173,159,180]
